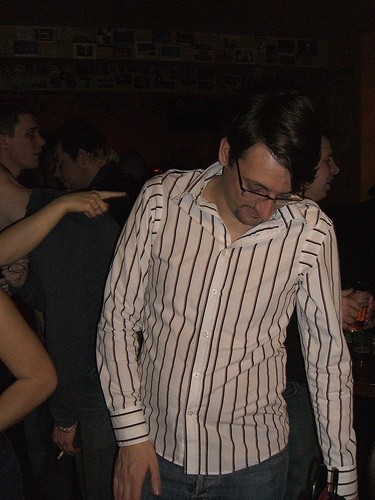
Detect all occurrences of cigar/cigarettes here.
[57,450,64,461]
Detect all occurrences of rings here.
[95,206,100,210]
[8,266,12,272]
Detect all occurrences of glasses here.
[235,157,305,206]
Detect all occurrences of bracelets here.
[55,423,78,433]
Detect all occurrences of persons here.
[95,86,360,500]
[0,94,146,500]
[283,130,375,500]
